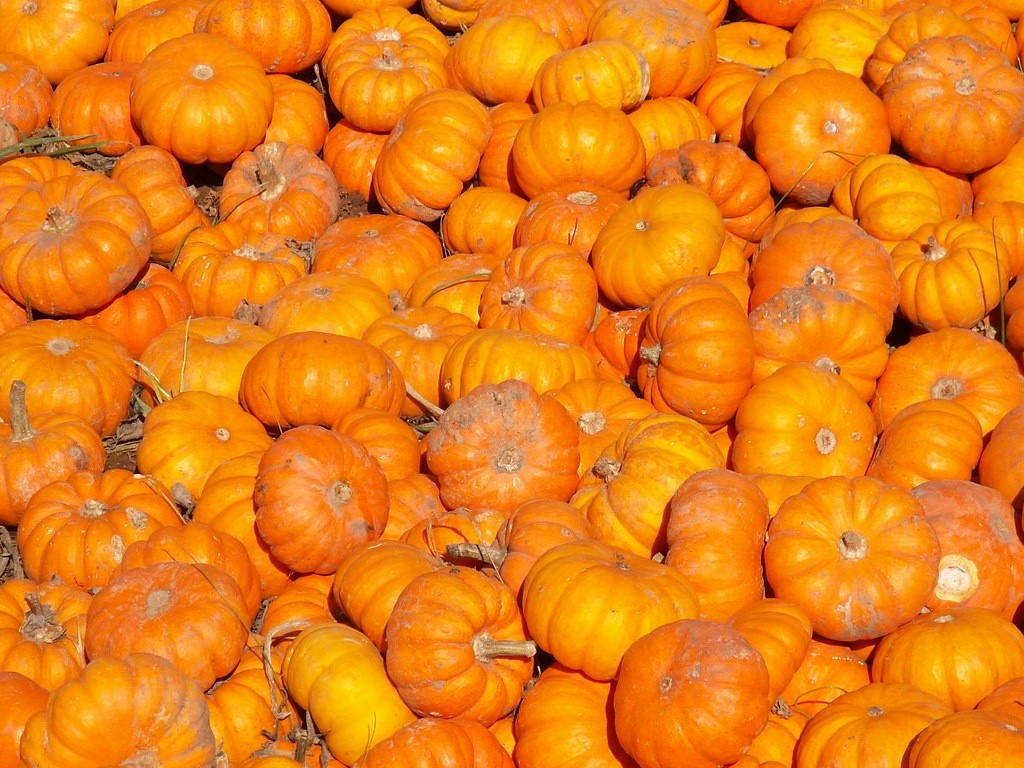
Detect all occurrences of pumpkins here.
[0,0,1024,768]
